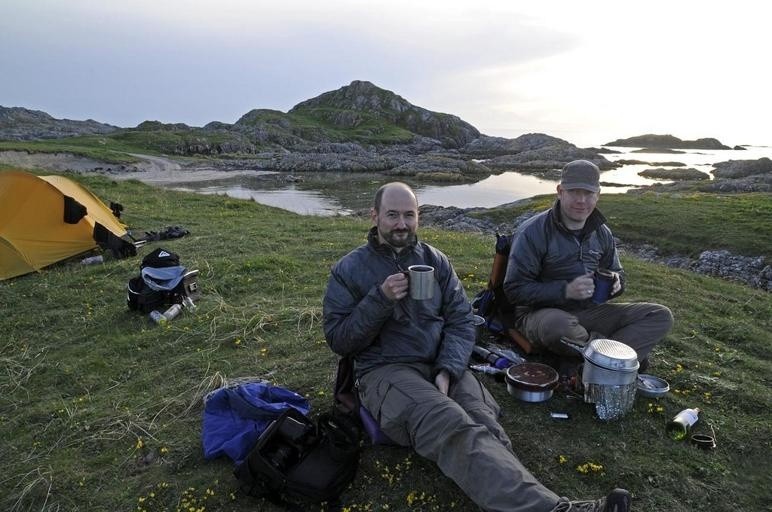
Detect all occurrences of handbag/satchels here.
[470,274,514,344]
[127,248,189,314]
[231,403,360,502]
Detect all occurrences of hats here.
[560,158,601,194]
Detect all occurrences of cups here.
[475,315,485,343]
[182,296,196,314]
[589,269,613,304]
[400,265,436,300]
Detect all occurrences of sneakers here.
[554,486,631,512]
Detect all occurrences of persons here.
[503,160,674,367]
[322,182,631,512]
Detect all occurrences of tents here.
[0,170,127,281]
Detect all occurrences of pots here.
[560,336,637,388]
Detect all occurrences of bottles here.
[150,310,167,326]
[163,304,181,321]
[470,345,506,370]
[80,255,104,264]
[665,406,700,439]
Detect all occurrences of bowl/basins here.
[690,433,714,447]
[637,374,670,399]
